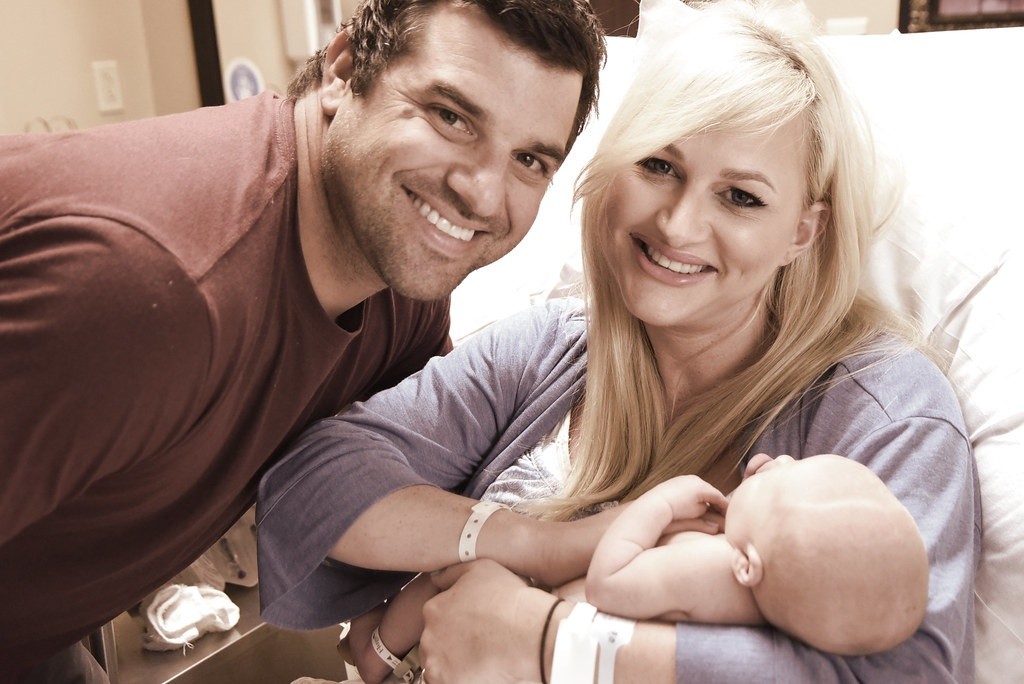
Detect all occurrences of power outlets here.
[93,59,124,112]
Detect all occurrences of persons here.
[0,0,607,684]
[337,453,929,684]
[255,7,984,684]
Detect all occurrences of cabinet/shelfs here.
[81,582,348,684]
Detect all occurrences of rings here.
[418,668,426,684]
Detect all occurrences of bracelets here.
[459,500,512,563]
[540,597,635,684]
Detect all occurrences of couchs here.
[450,27,1024,684]
